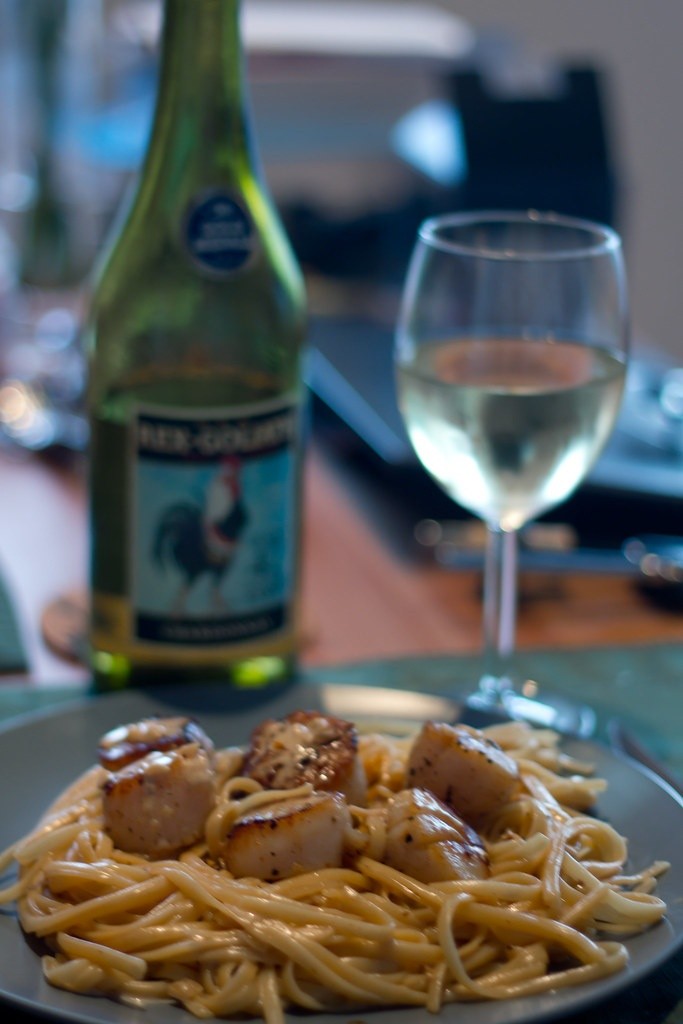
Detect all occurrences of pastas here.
[1,730,670,1024]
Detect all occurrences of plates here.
[1,683,682,1024]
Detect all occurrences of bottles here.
[84,1,312,712]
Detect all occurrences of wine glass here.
[394,210,633,740]
